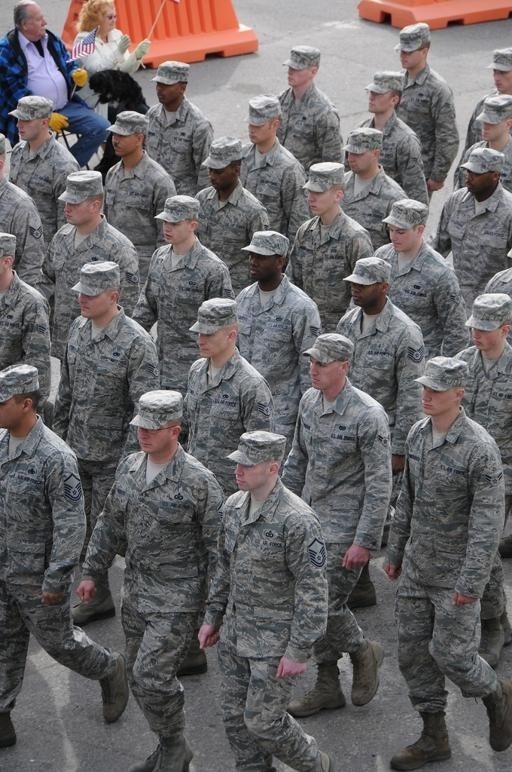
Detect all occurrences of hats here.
[302,162,345,193]
[58,171,104,205]
[458,148,506,175]
[188,298,240,337]
[71,259,121,297]
[475,94,512,125]
[1,231,17,258]
[129,390,185,432]
[485,47,512,72]
[303,332,354,365]
[1,134,8,166]
[342,256,390,286]
[282,46,322,70]
[395,21,431,53]
[152,62,190,86]
[106,111,150,137]
[464,293,512,332]
[8,95,54,121]
[241,231,291,256]
[0,360,39,403]
[364,70,404,95]
[246,93,282,127]
[153,195,200,224]
[382,198,428,231]
[201,136,243,170]
[227,431,286,465]
[413,357,469,393]
[342,128,383,154]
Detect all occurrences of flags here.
[64,28,96,63]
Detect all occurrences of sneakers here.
[311,750,334,772]
[498,534,512,557]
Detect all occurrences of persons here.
[385,22,459,207]
[274,46,341,171]
[1,0,110,170]
[453,47,511,187]
[72,0,151,186]
[343,69,427,207]
[145,61,212,194]
[1,96,512,772]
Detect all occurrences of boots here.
[391,710,451,769]
[178,650,208,676]
[128,746,159,772]
[350,640,385,706]
[475,609,512,669]
[67,571,116,626]
[0,710,16,748]
[288,661,346,716]
[99,651,130,723]
[480,677,511,752]
[347,562,376,610]
[153,734,193,771]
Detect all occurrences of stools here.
[54,125,100,171]
